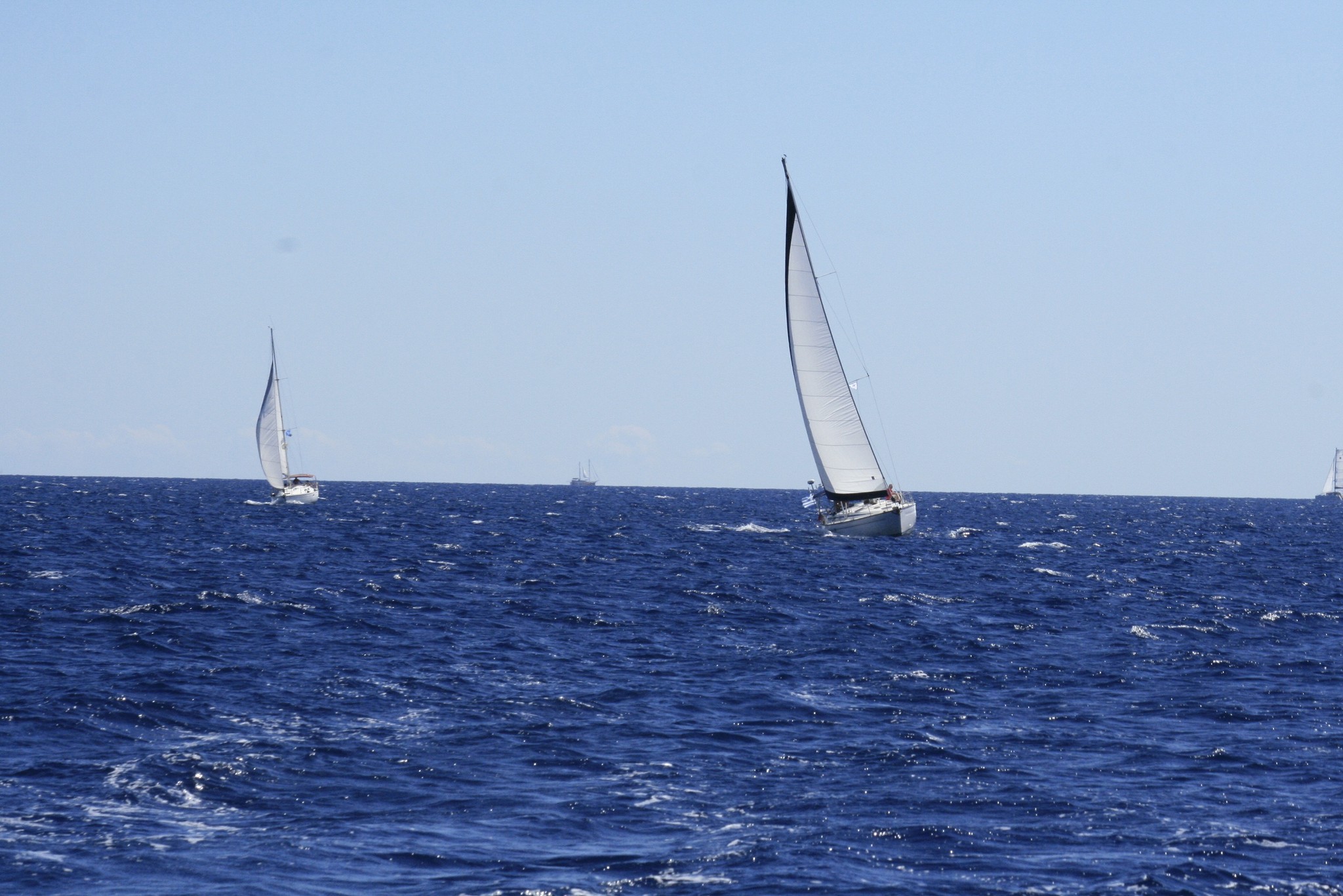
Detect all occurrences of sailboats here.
[255,325,320,504]
[779,153,915,535]
[569,460,596,487]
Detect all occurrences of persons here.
[886,484,900,502]
[305,480,311,486]
[292,477,302,485]
